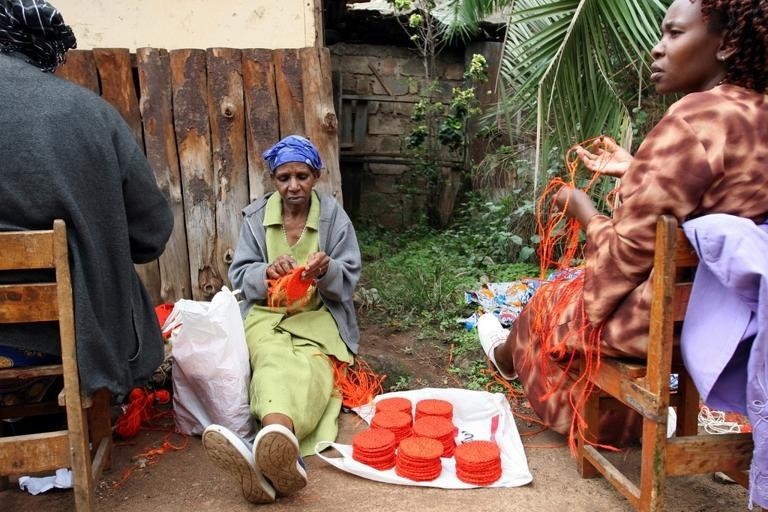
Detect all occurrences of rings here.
[319,267,321,273]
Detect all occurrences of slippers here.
[477,313,519,380]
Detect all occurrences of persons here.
[0,1,175,498]
[199,137,361,506]
[473,0,767,441]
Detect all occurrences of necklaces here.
[283,225,305,248]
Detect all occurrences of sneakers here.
[252,424,308,494]
[202,424,278,503]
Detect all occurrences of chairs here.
[577,213,768,511]
[2,219,117,512]
